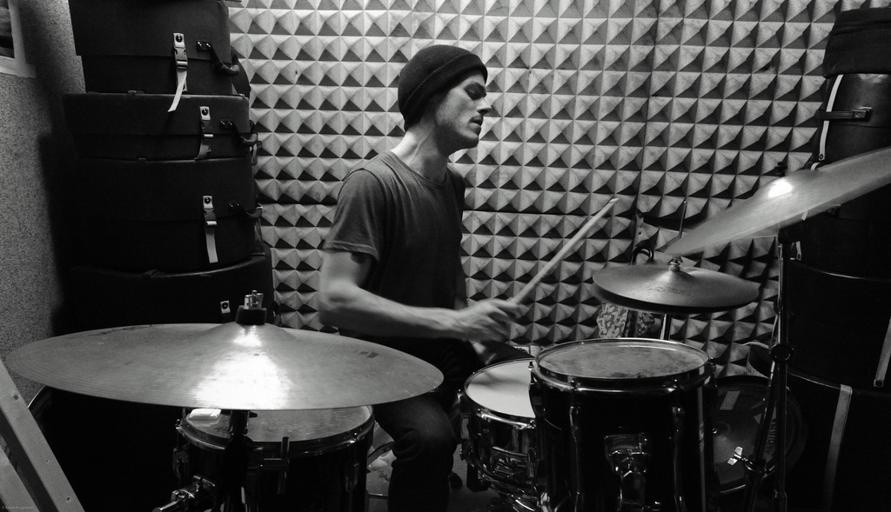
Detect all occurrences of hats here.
[398,44,489,133]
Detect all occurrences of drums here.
[528,336,719,512]
[174,406,376,512]
[459,357,536,500]
[713,375,806,512]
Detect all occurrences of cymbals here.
[7,290,444,410]
[665,146,891,260]
[593,263,758,313]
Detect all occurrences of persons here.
[315,44,528,512]
